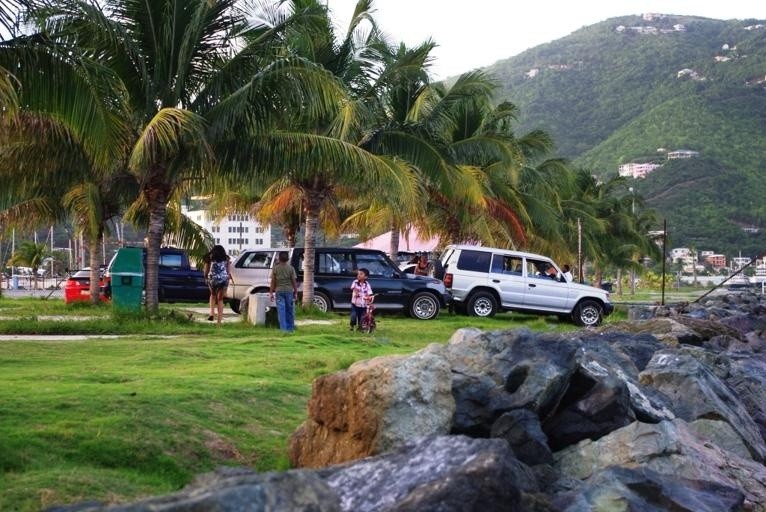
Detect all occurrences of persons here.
[561,264,572,282]
[268,251,298,332]
[202,244,235,325]
[348,268,375,331]
[413,254,435,279]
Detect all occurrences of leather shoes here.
[207,316,212,321]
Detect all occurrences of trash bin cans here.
[600,282,612,293]
[108,247,146,316]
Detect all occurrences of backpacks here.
[207,259,228,288]
